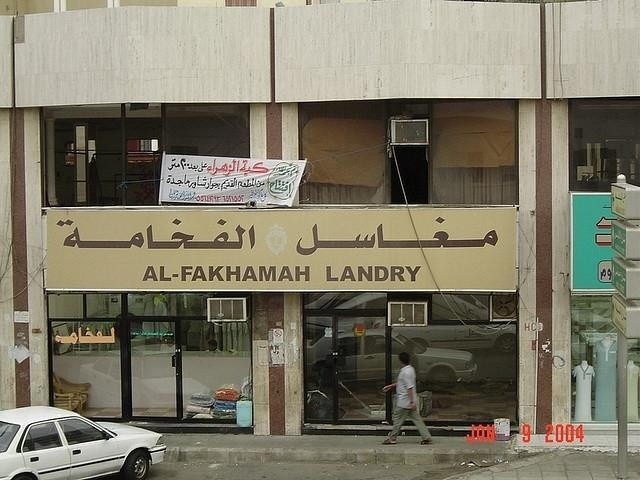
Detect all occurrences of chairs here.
[53,371,91,417]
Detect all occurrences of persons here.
[381,351,433,445]
[205,339,222,352]
[159,331,175,353]
[572,336,640,423]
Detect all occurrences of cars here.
[0,405,165,479]
[570,307,617,374]
[302,293,517,383]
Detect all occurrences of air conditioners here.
[390,119,429,145]
[387,300,429,327]
[206,295,251,323]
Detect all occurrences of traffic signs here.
[609,218,640,260]
[609,294,639,340]
[610,256,640,301]
[610,182,640,222]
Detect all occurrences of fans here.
[490,295,518,322]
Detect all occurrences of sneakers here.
[420,440,433,445]
[383,439,397,444]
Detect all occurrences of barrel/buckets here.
[236,401,252,428]
[494,418,510,441]
